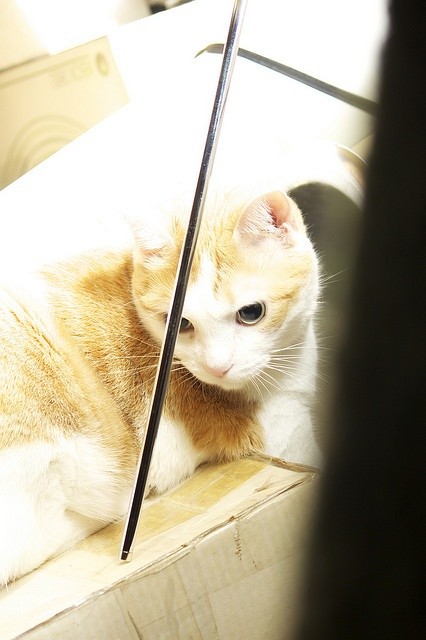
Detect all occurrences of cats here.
[0,189,331,590]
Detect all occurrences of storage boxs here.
[0,450,322,640]
